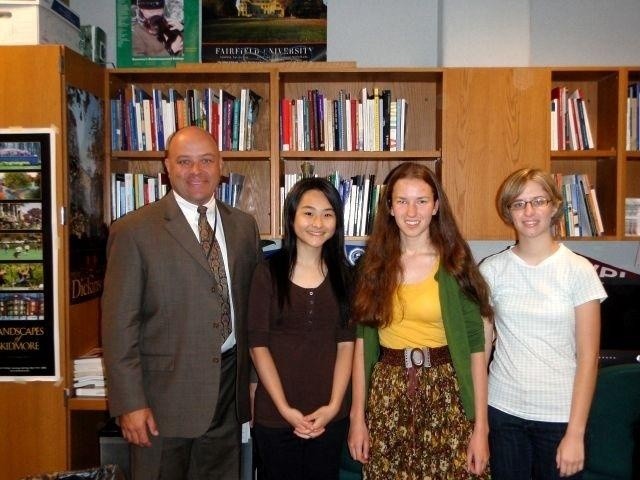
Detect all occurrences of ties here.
[197,205,233,346]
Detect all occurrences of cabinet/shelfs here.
[102,66,639,244]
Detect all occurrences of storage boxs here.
[0,0,83,57]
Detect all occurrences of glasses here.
[508,197,552,211]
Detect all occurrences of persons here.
[465,167,609,479]
[132,8,184,58]
[97,123,268,480]
[244,176,361,479]
[344,163,494,480]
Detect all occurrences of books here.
[279,87,407,152]
[549,80,640,241]
[110,84,263,152]
[71,346,110,402]
[111,172,244,222]
[279,170,387,237]
